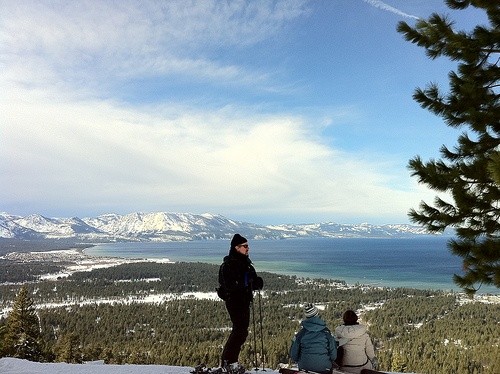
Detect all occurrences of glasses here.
[239,244,249,248]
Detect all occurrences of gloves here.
[253,277,263,291]
[305,303,319,319]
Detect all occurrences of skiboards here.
[359,368,388,374]
[279,368,322,374]
[191,366,247,374]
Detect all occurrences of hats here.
[231,234,247,246]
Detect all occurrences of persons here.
[218,233,263,373]
[334,310,379,374]
[291,303,338,374]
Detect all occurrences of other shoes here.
[221,359,245,374]
